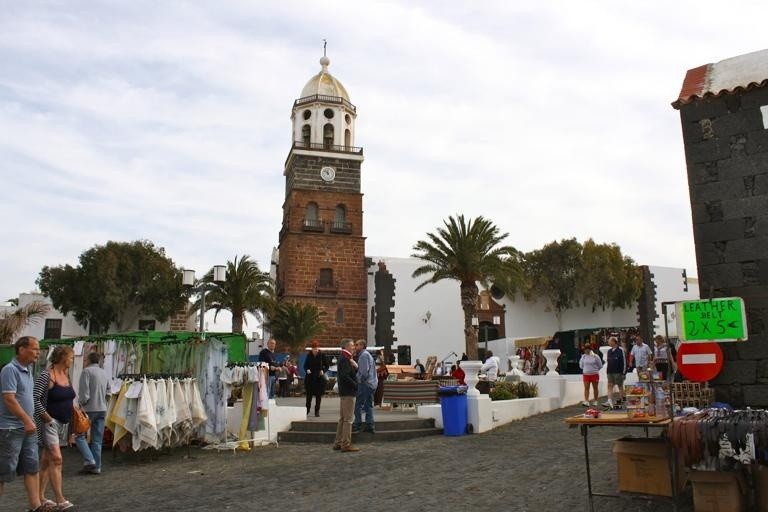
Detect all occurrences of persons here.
[304,340,329,417]
[478,350,499,386]
[374,358,389,407]
[0,336,55,512]
[352,338,378,434]
[579,336,677,407]
[414,359,465,387]
[75,352,112,475]
[333,338,360,452]
[259,338,298,399]
[33,346,76,511]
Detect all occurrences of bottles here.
[655,384,666,417]
[647,392,655,416]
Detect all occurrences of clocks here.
[320,167,335,181]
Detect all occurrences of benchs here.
[381,377,459,413]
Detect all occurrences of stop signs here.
[675,338,723,382]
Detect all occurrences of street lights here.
[471,315,501,358]
[181,263,227,334]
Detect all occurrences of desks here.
[560,409,680,512]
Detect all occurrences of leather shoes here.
[352,426,376,434]
[333,444,360,451]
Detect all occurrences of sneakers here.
[78,464,100,474]
[582,401,625,409]
[28,499,74,512]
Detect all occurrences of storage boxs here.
[610,433,674,498]
[681,467,748,512]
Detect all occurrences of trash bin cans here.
[436,386,473,436]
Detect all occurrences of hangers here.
[223,360,268,370]
[115,372,196,385]
[80,334,224,346]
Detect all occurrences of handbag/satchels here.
[69,405,90,433]
